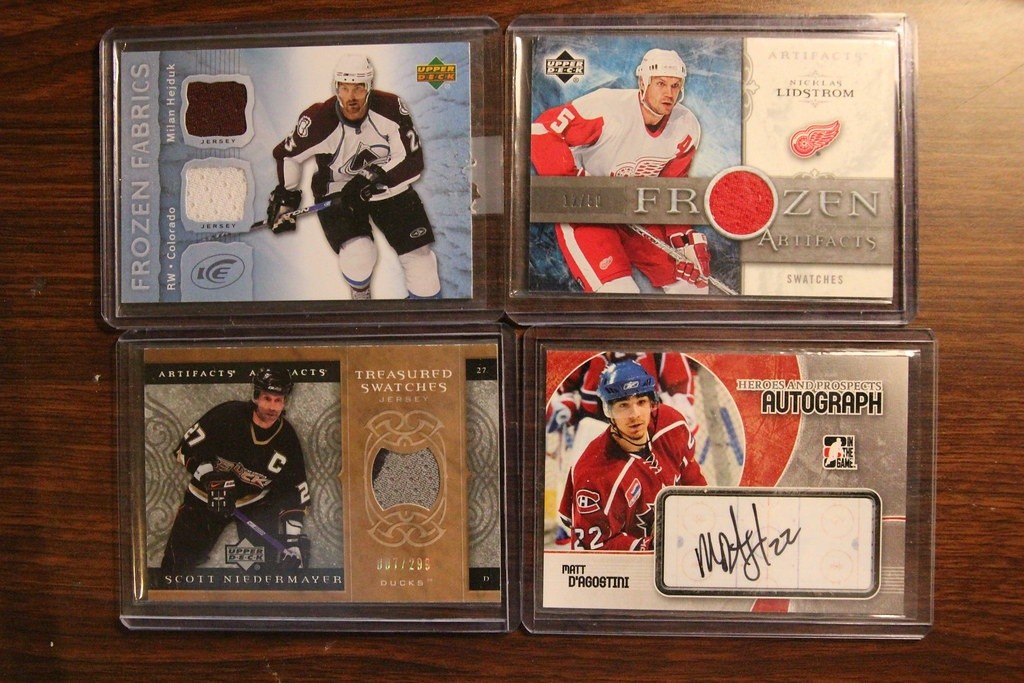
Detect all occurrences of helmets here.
[334,53,374,94]
[635,48,687,87]
[598,359,655,402]
[251,367,293,401]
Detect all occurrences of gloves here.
[275,537,301,572]
[670,230,711,289]
[207,479,236,517]
[341,163,388,207]
[267,184,301,234]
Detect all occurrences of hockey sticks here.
[222,504,308,569]
[184,188,370,241]
[625,222,741,296]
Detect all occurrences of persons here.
[266,55,441,300]
[546,351,710,551]
[530,49,710,295]
[157,363,311,569]
